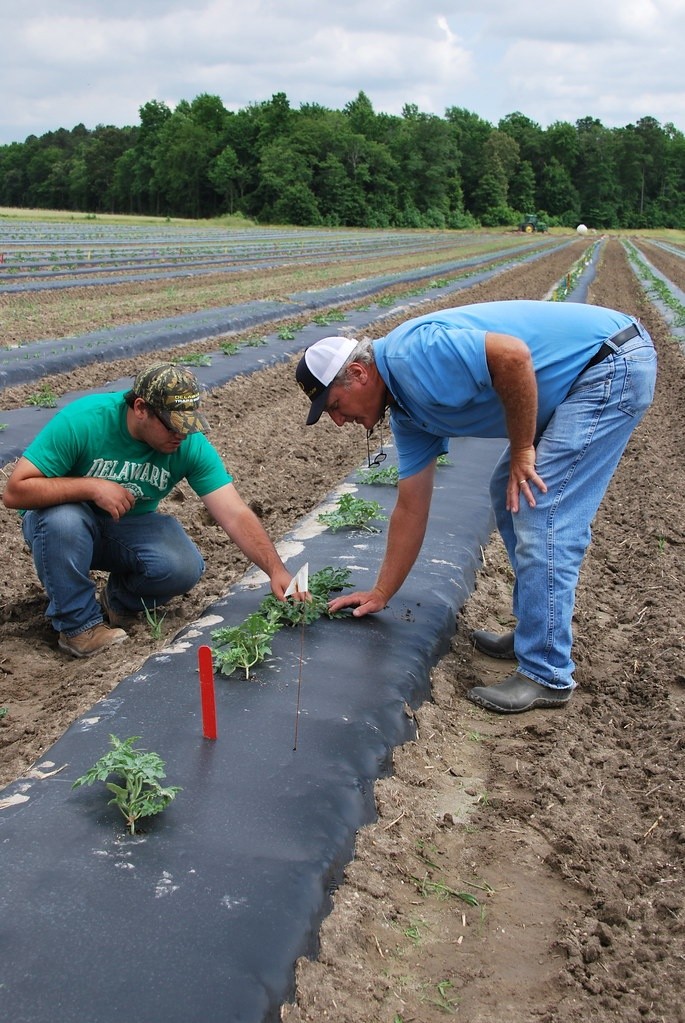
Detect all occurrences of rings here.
[519,480,527,485]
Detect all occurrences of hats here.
[133,362,210,433]
[295,337,358,425]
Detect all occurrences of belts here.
[579,323,644,374]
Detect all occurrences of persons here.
[293,299,657,715]
[3,361,315,658]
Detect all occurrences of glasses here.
[367,435,386,471]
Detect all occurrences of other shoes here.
[471,630,517,660]
[466,671,571,713]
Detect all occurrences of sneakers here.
[57,621,129,657]
[98,585,137,630]
